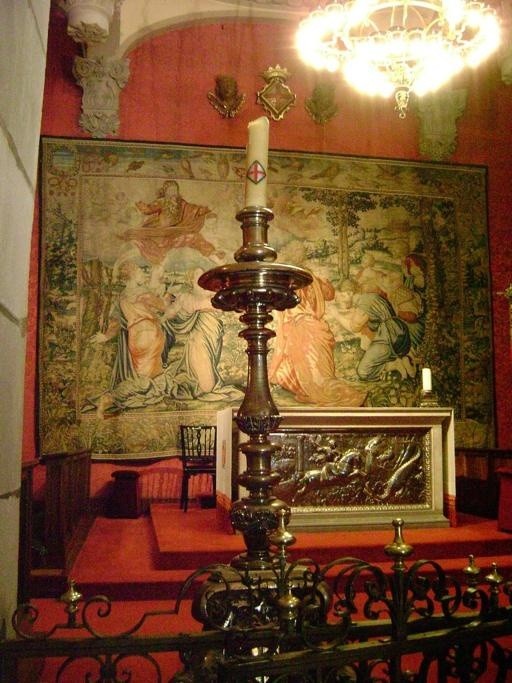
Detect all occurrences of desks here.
[215,405,460,537]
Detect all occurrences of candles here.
[243,115,273,207]
[422,366,432,390]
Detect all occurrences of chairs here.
[178,424,217,512]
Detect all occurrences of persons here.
[330,242,428,380]
[102,262,222,397]
[125,179,222,271]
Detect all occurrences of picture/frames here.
[34,134,500,459]
